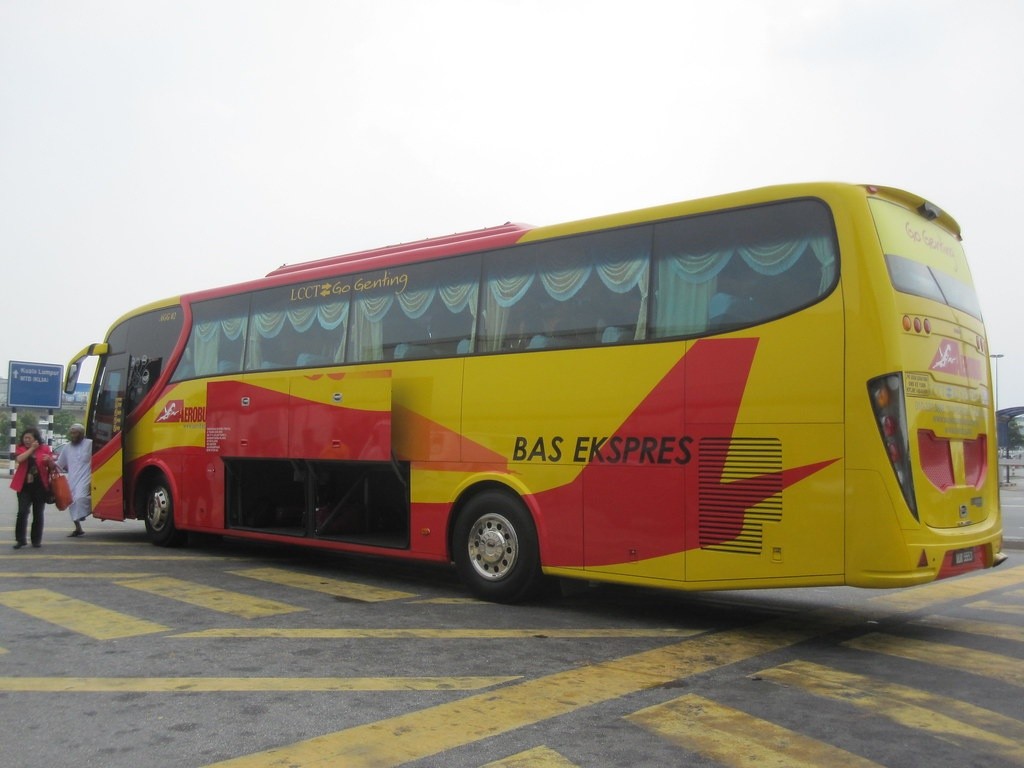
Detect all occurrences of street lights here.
[989,353,1004,420]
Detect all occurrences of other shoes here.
[13,541,27,549]
[31,540,41,547]
[67,531,85,537]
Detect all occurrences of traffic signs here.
[8,360,62,408]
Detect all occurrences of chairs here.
[296,352,330,365]
[394,345,437,360]
[456,338,470,352]
[260,360,286,368]
[600,325,634,343]
[707,292,742,329]
[526,334,574,348]
[218,361,240,373]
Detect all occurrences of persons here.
[10,427,56,549]
[56,423,106,537]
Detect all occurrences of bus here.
[62,188,1010,607]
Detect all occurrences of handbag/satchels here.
[50,465,73,510]
[45,467,55,504]
[303,480,350,535]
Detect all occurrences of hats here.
[69,424,85,433]
